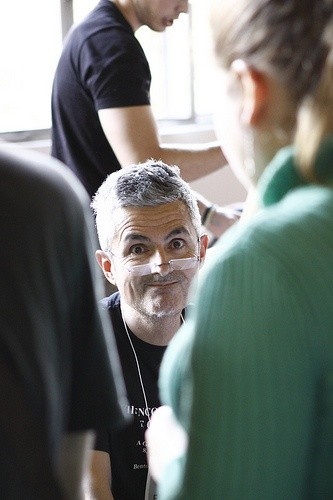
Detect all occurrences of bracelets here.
[201,202,214,228]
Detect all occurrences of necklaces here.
[119,296,187,423]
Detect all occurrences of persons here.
[51,0,243,239]
[89,158,209,500]
[0,140,133,500]
[159,0,333,500]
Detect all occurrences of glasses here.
[107,237,199,276]
